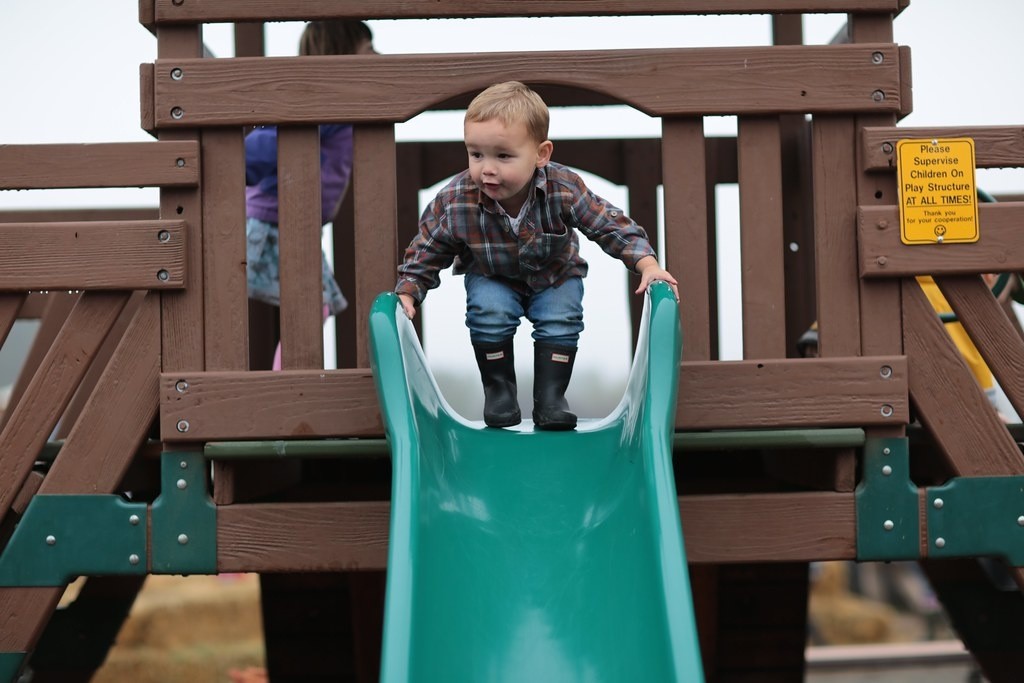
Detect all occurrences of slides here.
[385,365,706,683]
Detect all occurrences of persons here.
[394,82,680,429]
[246,17,381,370]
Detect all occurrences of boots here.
[533,341,578,429]
[471,337,521,426]
